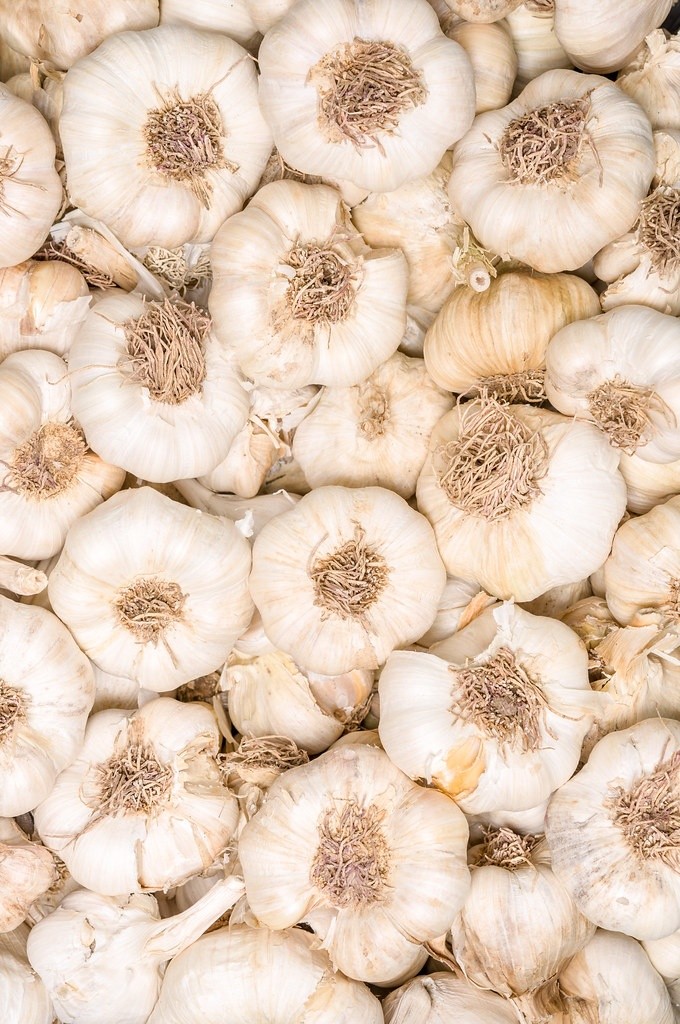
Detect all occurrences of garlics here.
[0,0,680,1024]
